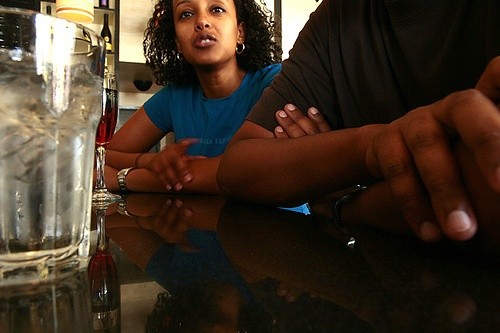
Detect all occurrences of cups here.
[0,7,106,287]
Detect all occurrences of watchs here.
[325,180,368,227]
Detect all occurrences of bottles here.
[100,13,112,54]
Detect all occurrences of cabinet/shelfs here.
[95,0,281,152]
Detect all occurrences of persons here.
[93,0,312,215]
[105,194,283,333]
[217,201,500,333]
[216,0,500,244]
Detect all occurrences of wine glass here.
[91,74,121,201]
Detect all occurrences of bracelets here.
[117,167,135,193]
